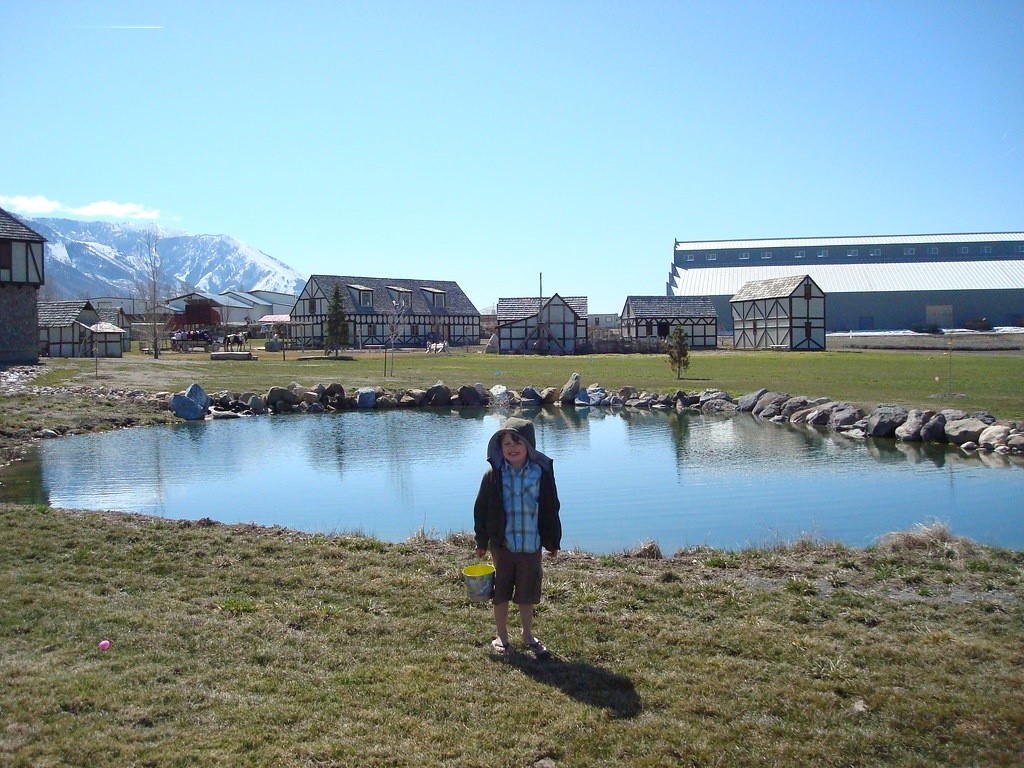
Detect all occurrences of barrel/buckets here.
[462,555,495,603]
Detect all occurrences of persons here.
[473,417,562,656]
[272,331,280,342]
[171,328,211,342]
[425,338,450,354]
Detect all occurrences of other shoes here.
[491,637,515,656]
[525,636,547,656]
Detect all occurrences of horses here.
[223,332,249,351]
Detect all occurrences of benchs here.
[770,345,789,351]
[365,344,385,353]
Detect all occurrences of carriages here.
[171,332,251,352]
